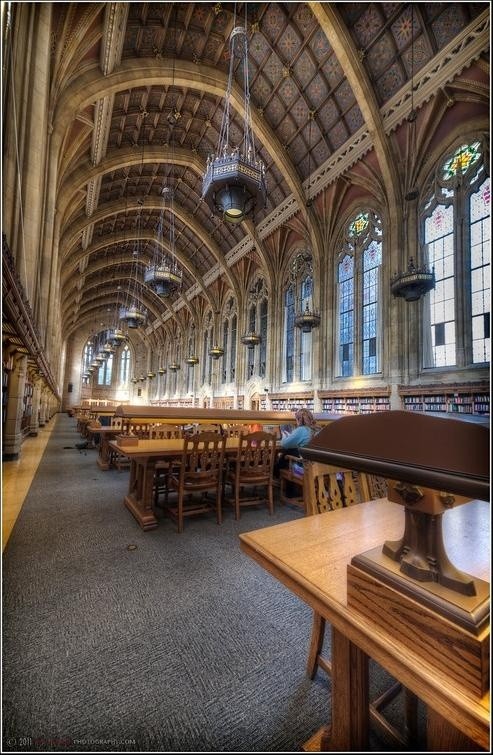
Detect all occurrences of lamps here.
[143,7,184,298]
[78,232,129,385]
[208,218,225,360]
[390,7,435,302]
[241,222,262,350]
[120,196,148,330]
[203,5,268,223]
[130,267,201,385]
[293,112,321,333]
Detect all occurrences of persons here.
[274,408,324,488]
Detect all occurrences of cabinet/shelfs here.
[148,379,489,415]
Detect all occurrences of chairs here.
[69,402,302,528]
[299,459,380,681]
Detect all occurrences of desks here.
[238,494,488,753]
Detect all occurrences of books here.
[321,398,390,416]
[404,396,490,416]
[260,399,313,412]
